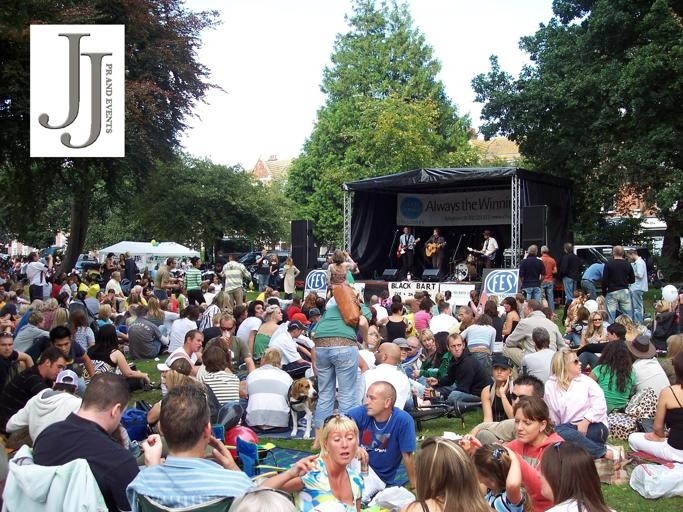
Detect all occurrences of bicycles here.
[405,392,481,434]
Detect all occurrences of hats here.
[491,356,514,368]
[286,306,322,329]
[56,369,78,387]
[624,335,655,359]
[392,338,412,351]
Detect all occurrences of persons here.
[0,222,682,512]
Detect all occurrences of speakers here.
[292,220,313,280]
[422,270,440,281]
[523,205,548,257]
[382,269,398,281]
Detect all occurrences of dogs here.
[288,377,318,440]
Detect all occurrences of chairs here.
[133,489,234,512]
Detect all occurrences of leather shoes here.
[606,445,625,461]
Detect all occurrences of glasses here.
[0,332,15,338]
[241,488,294,505]
[506,394,535,400]
[554,441,562,464]
[221,324,234,331]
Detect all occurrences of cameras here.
[45,256,49,261]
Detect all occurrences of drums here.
[454,263,477,281]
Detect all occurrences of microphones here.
[461,234,466,237]
[432,233,436,236]
[394,229,399,234]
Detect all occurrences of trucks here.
[572,216,683,292]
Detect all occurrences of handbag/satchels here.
[122,399,157,441]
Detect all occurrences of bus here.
[214,237,258,269]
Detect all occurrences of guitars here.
[467,247,496,260]
[426,242,446,256]
[397,237,420,257]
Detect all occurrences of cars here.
[41,247,64,258]
[215,251,332,289]
[74,254,98,271]
